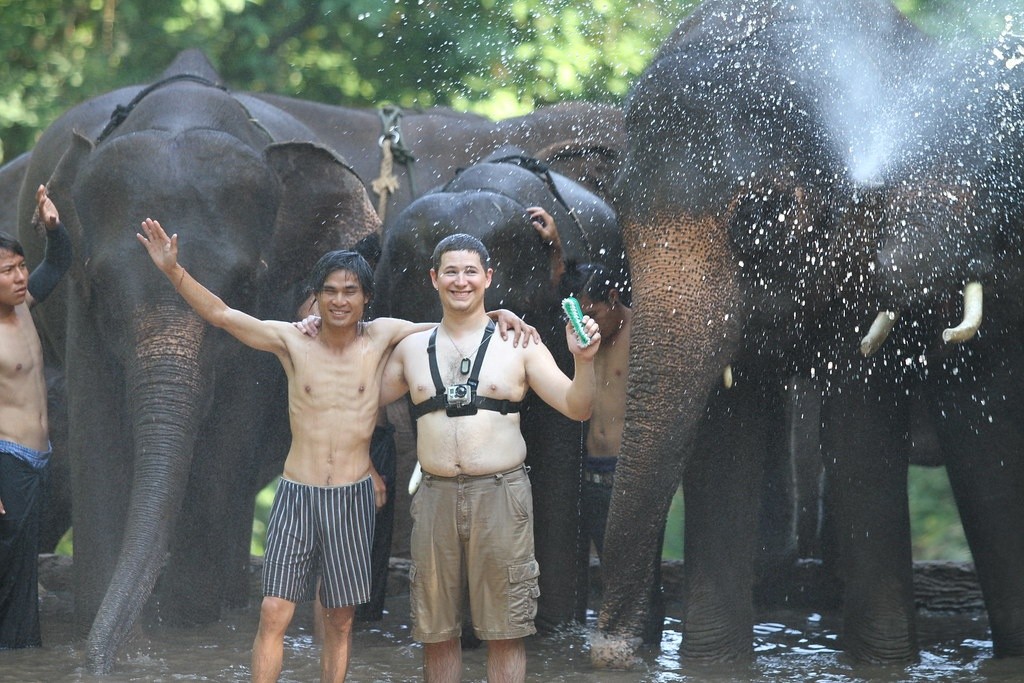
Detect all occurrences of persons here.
[291,234,602,683]
[525,205,664,647]
[0,186,75,648]
[137,217,542,683]
[294,292,399,638]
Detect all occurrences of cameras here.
[448,385,471,407]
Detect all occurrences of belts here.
[584,471,614,487]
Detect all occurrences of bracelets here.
[174,267,185,292]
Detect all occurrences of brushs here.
[561,296,593,350]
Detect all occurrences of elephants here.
[2,1,1023,683]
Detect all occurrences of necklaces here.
[440,316,499,374]
